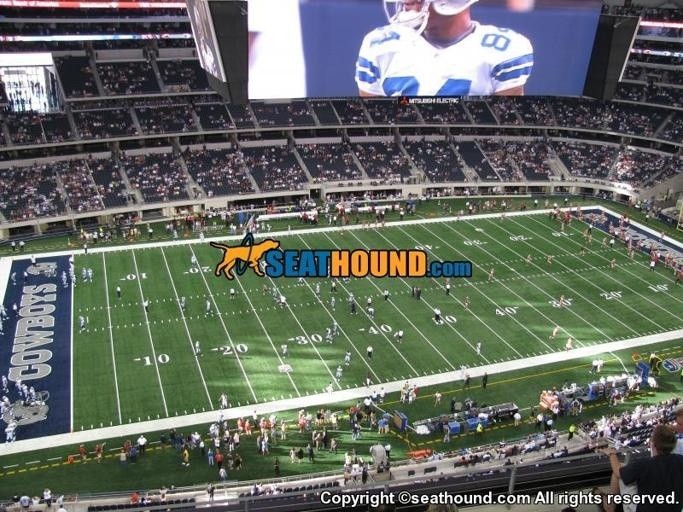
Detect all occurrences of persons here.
[353,0,534,97]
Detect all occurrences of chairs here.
[84,402,681,512]
[0,0,683,235]
